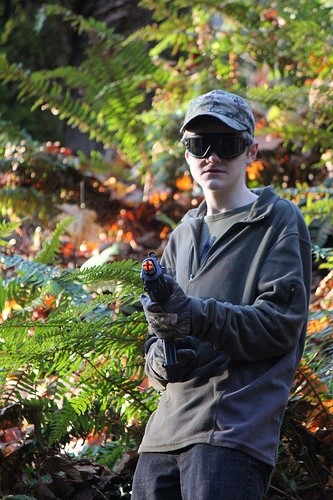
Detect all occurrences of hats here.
[179,89,255,144]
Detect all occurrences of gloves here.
[139,274,190,339]
[145,345,198,391]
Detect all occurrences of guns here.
[140,249,180,368]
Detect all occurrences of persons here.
[136,88,312,500]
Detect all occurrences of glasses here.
[184,134,252,161]
